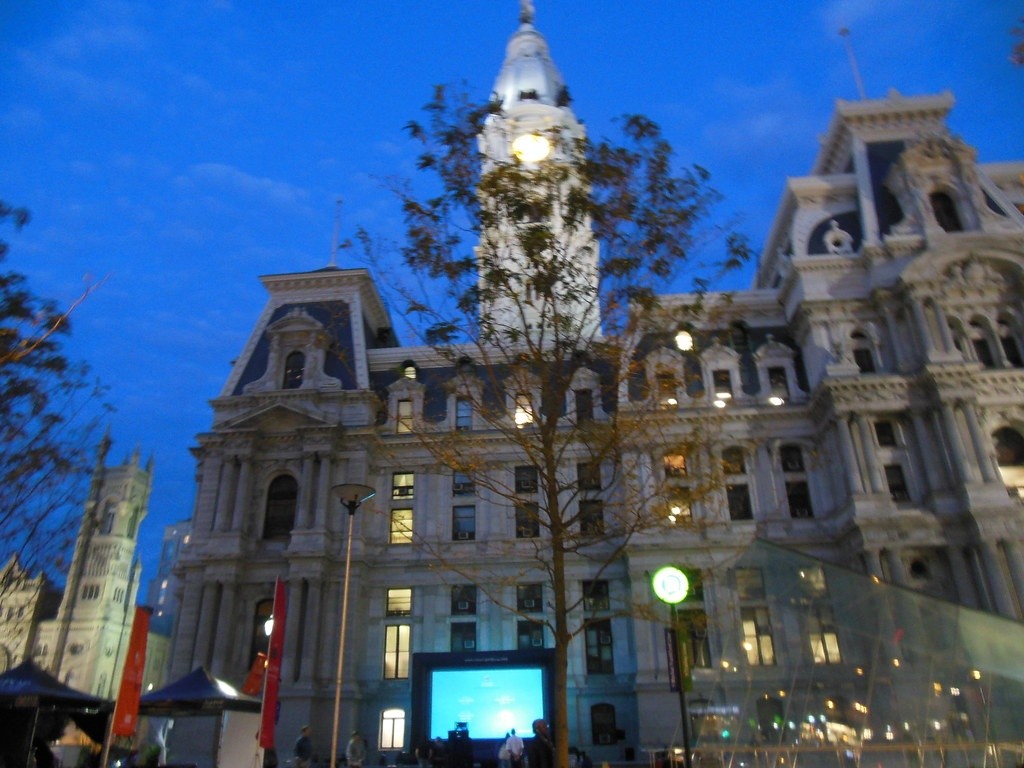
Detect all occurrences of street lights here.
[325,480,377,767]
[650,563,695,768]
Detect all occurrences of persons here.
[498,719,556,768]
[347,731,366,768]
[415,736,433,768]
[294,726,312,768]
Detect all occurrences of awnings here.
[0,654,265,768]
